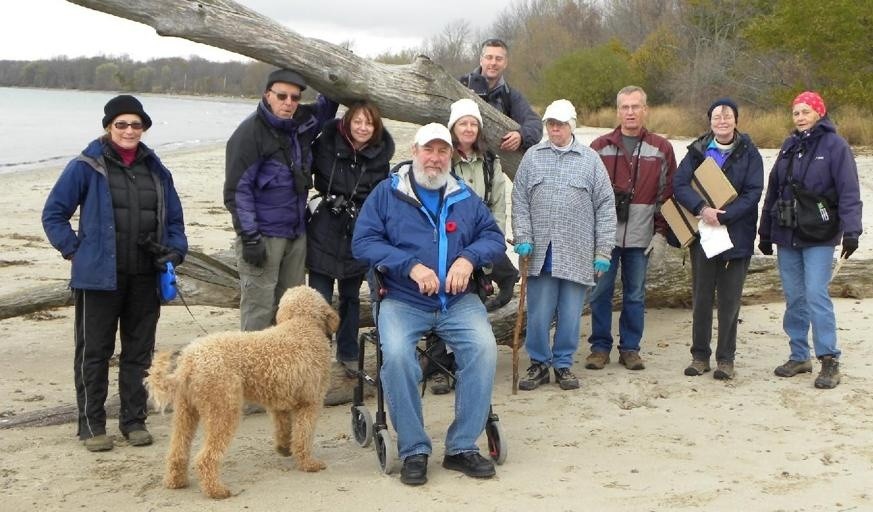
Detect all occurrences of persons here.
[39,91,186,453]
[586,83,680,370]
[430,97,509,393]
[671,97,765,380]
[462,39,542,154]
[757,91,862,389]
[222,69,339,332]
[350,121,509,485]
[510,97,616,390]
[309,98,395,382]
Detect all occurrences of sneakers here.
[815,356,840,389]
[431,370,450,394]
[685,357,710,376]
[442,451,496,477]
[774,359,812,377]
[400,453,427,483]
[619,351,645,370]
[520,358,550,390]
[553,367,579,390]
[585,351,610,369]
[713,360,734,379]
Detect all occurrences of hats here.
[793,91,826,118]
[265,69,306,94]
[415,122,453,149]
[542,99,577,123]
[448,99,483,132]
[708,98,738,119]
[103,95,152,132]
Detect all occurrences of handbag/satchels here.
[615,190,631,222]
[776,198,797,229]
[792,185,839,241]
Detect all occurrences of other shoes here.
[338,358,359,379]
[86,433,112,451]
[128,429,152,445]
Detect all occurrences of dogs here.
[140,284,341,500]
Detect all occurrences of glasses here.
[268,88,302,102]
[112,121,144,130]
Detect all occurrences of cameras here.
[325,194,357,220]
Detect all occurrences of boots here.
[493,253,520,306]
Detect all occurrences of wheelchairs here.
[347,259,506,474]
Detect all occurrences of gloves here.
[593,259,611,273]
[330,74,347,89]
[644,233,668,268]
[137,231,181,271]
[841,239,858,259]
[514,243,534,257]
[758,239,773,255]
[242,235,268,268]
